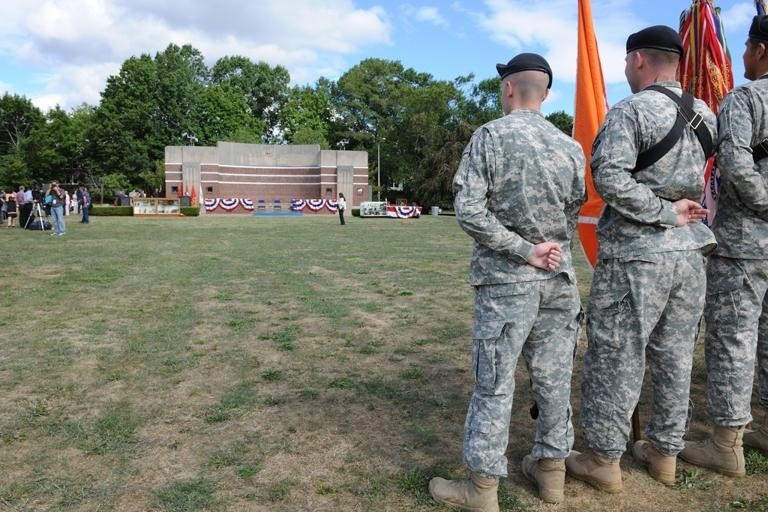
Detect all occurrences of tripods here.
[23,202,47,232]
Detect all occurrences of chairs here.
[257,199,264,211]
[272,200,281,211]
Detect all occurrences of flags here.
[191,185,197,206]
[679,0,734,240]
[574,0,609,274]
[177,184,182,198]
[186,184,190,195]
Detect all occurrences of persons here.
[338,193,346,225]
[0,179,90,238]
[565,25,717,494]
[675,16,768,480]
[428,51,587,512]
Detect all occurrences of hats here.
[496,52,553,90]
[625,24,685,58]
[748,14,768,42]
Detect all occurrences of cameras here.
[51,184,58,190]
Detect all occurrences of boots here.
[564,447,625,495]
[633,438,677,486]
[522,452,567,504]
[678,422,748,477]
[428,474,502,512]
[738,413,767,454]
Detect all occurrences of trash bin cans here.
[116,194,129,206]
[432,206,439,215]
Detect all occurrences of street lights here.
[376,137,387,204]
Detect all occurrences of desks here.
[384,205,421,218]
